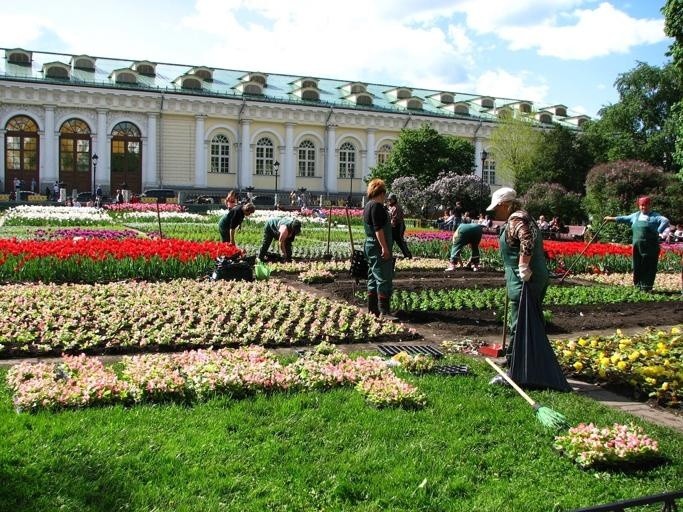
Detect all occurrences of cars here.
[77,192,94,202]
[20,191,38,201]
[142,188,176,198]
[252,196,273,205]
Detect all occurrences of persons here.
[536,214,560,239]
[46,180,123,207]
[363,178,412,318]
[225,190,237,210]
[218,203,256,246]
[659,224,683,242]
[258,217,301,263]
[485,187,550,369]
[439,202,491,231]
[445,224,483,271]
[603,197,670,294]
[290,189,308,212]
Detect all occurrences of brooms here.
[484,353,565,431]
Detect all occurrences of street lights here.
[91,154,98,194]
[348,166,354,201]
[479,149,488,219]
[273,160,280,206]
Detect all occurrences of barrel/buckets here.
[254,264,271,280]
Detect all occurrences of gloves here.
[518,263,533,282]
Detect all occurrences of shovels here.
[323,208,332,262]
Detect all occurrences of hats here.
[485,187,517,211]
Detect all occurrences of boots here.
[366,292,400,322]
